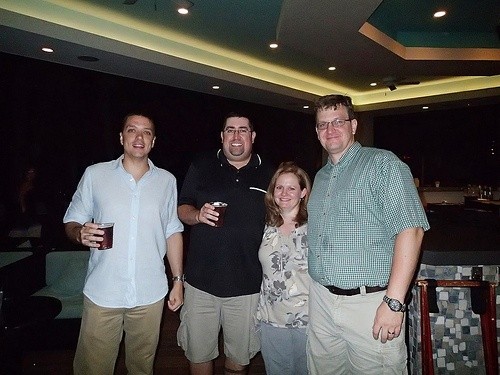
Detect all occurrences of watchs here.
[172,274,187,283]
[383,296,406,312]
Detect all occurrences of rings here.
[388,331,394,334]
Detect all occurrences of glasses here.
[317,119,350,129]
[225,129,252,134]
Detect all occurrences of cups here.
[463,184,492,208]
[434,181,440,188]
[209,202,226,227]
[94,222,114,251]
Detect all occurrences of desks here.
[419,185,466,208]
[0,295,62,375]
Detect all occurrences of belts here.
[326,284,387,296]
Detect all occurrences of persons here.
[63,110,185,375]
[256,160,311,375]
[307,94,431,375]
[177,113,269,375]
